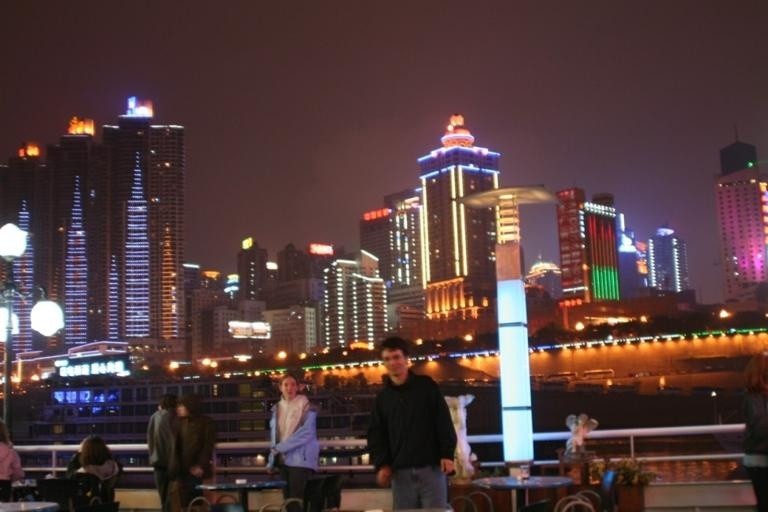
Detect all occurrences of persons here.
[0,418,26,502]
[159,392,218,511]
[266,373,322,510]
[147,394,176,495]
[64,431,122,511]
[737,352,767,512]
[366,335,458,509]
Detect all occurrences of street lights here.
[0,221,66,446]
[450,181,564,484]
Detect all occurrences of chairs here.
[0,458,656,512]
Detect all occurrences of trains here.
[10,375,376,488]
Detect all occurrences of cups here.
[517,464,531,485]
